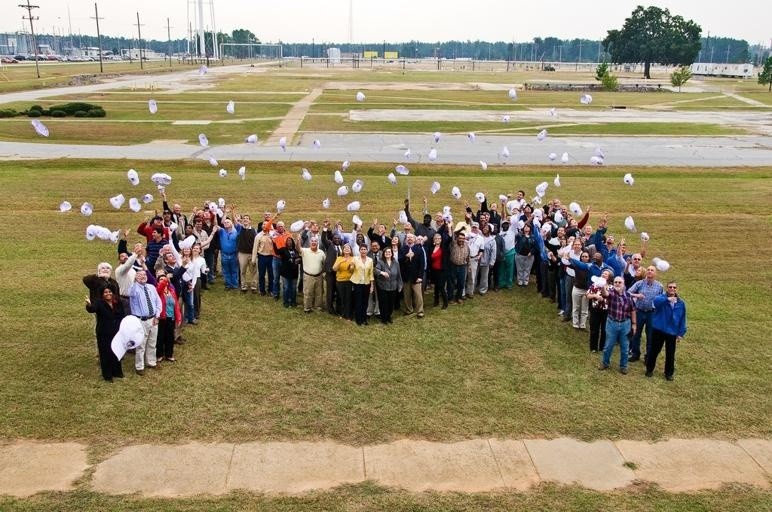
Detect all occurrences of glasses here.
[670,287,677,290]
[634,259,642,261]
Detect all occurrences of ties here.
[144,286,155,316]
[375,252,377,264]
[337,246,340,256]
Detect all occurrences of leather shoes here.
[599,366,610,371]
[646,371,654,378]
[622,368,629,375]
[667,375,675,382]
[136,370,145,375]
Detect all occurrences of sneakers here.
[157,357,164,362]
[523,282,529,286]
[573,325,580,329]
[518,282,523,285]
[166,357,174,362]
[580,325,586,329]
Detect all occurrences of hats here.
[149,99,157,113]
[177,234,195,250]
[499,89,634,206]
[151,173,171,195]
[625,215,671,271]
[472,222,480,230]
[111,315,146,363]
[199,65,207,75]
[110,193,153,212]
[226,100,234,113]
[199,134,320,181]
[277,200,286,214]
[387,131,489,224]
[356,91,365,101]
[323,161,364,226]
[60,201,120,244]
[209,198,226,219]
[591,275,607,288]
[32,119,51,139]
[290,220,304,232]
[511,202,584,235]
[170,223,178,231]
[128,169,139,185]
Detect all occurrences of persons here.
[399,190,536,318]
[203,200,418,325]
[533,199,686,383]
[83,192,225,383]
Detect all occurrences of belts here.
[609,316,625,322]
[135,315,156,320]
[305,272,322,277]
